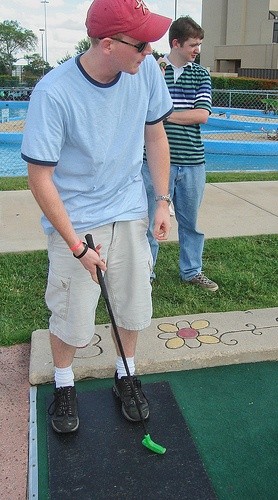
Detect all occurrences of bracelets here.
[70,240,89,261]
[155,195,172,204]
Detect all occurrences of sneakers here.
[190,274,219,293]
[111,369,151,422]
[47,376,80,434]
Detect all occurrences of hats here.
[85,0,173,43]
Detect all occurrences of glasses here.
[107,37,148,54]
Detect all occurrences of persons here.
[141,16,218,292]
[20,0,174,433]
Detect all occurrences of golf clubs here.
[85,234,166,454]
